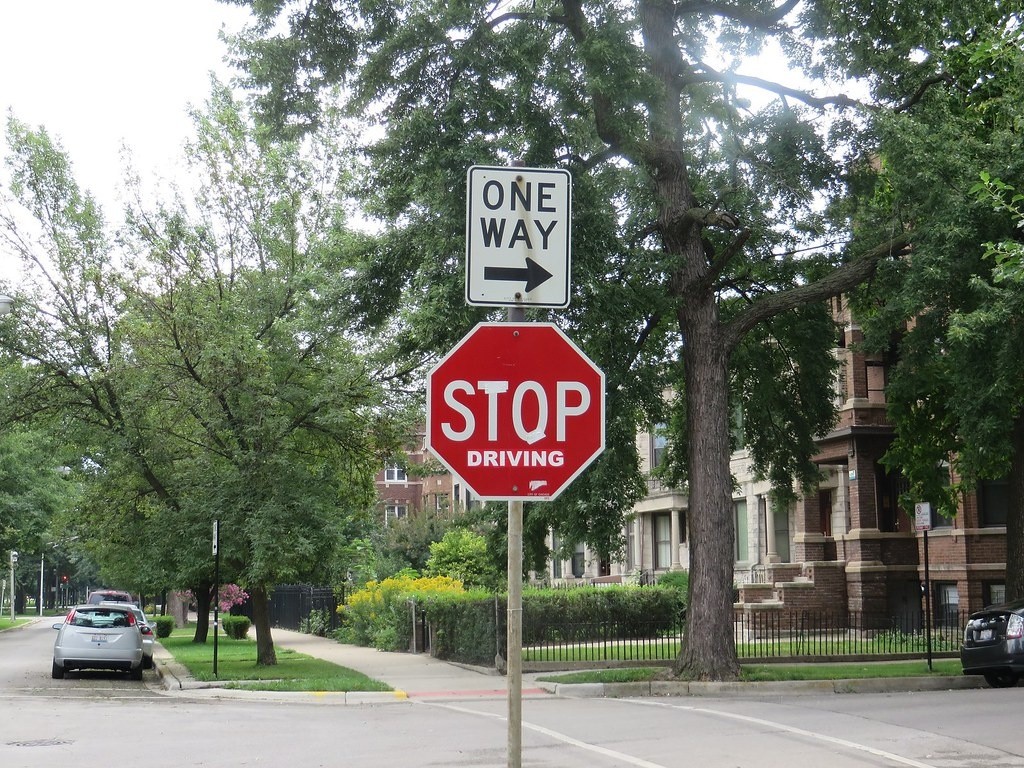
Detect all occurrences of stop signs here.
[426,322,607,502]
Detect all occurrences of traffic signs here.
[463,163,575,311]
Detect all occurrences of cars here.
[99,603,157,669]
[51,604,152,680]
[957,598,1024,690]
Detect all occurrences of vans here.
[86,589,132,606]
